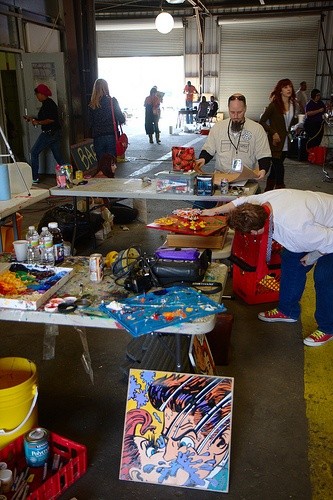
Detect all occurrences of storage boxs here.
[0,357,37,450]
[228,207,284,304]
[0,426,88,500]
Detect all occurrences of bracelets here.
[317,250,327,256]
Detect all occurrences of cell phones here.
[23,115,30,121]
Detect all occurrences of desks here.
[0,186,51,252]
[175,110,199,130]
[51,175,260,234]
[0,253,230,401]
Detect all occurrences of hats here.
[37,84,52,96]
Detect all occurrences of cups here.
[12,240,28,261]
[298,114,305,124]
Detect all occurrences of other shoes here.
[150,139,161,143]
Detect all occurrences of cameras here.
[123,273,152,294]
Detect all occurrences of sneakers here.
[258,308,298,322]
[304,330,332,346]
[32,179,39,184]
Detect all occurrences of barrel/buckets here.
[24,428,49,467]
[0,356,38,448]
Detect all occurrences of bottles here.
[0,164,10,201]
[39,226,55,265]
[25,226,40,265]
[0,469,13,493]
[55,164,83,189]
[221,178,229,193]
[48,222,64,262]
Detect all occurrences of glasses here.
[315,95,320,96]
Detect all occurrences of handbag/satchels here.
[116,133,128,156]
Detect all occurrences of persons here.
[304,89,327,147]
[294,82,308,124]
[206,96,219,117]
[197,96,209,126]
[259,78,297,193]
[25,82,66,184]
[87,78,126,162]
[143,87,161,143]
[199,188,333,347]
[153,85,163,121]
[189,92,272,209]
[92,152,139,223]
[182,81,198,124]
